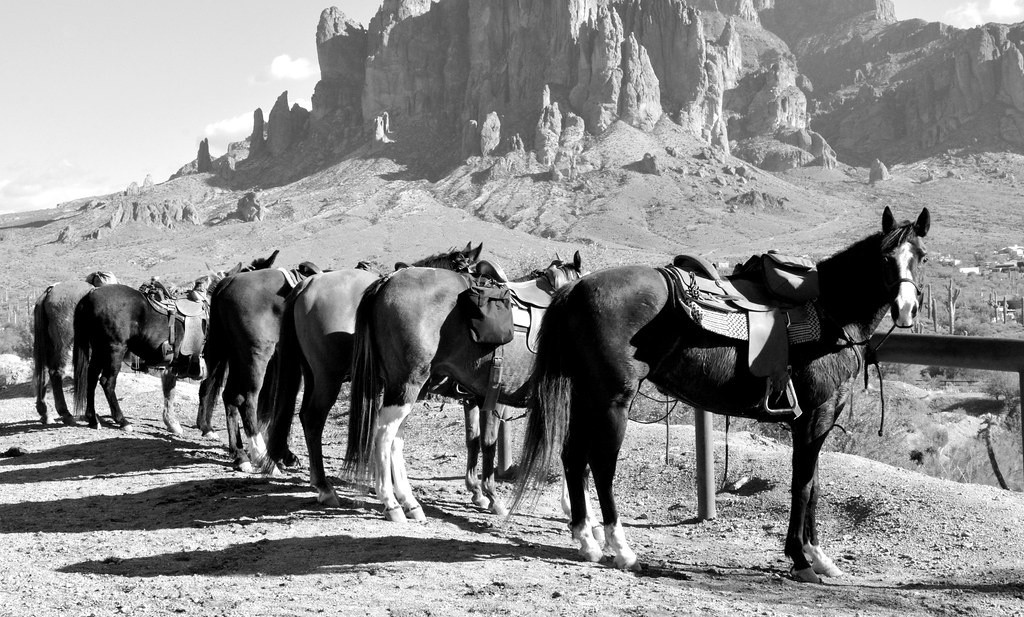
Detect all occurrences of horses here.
[28,241,483,506]
[336,250,592,523]
[500,205,931,583]
